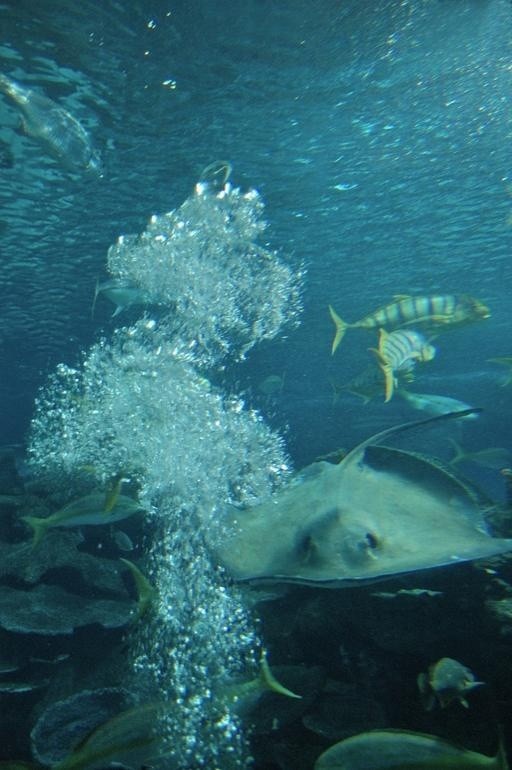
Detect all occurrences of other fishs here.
[0,368,512,770]
